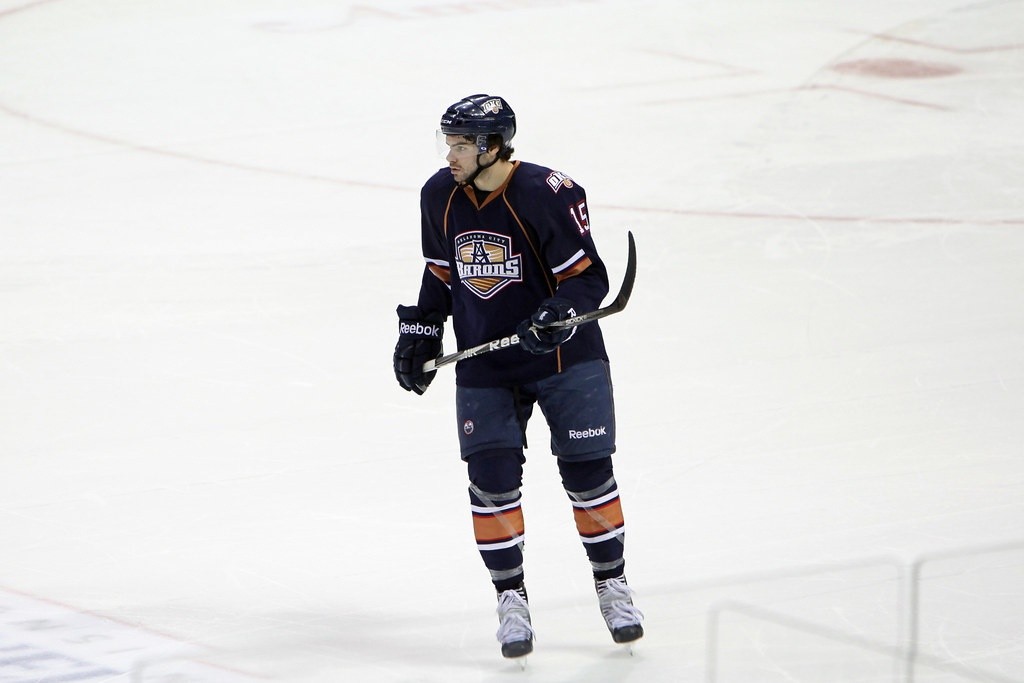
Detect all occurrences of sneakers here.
[496,580,536,671]
[594,572,644,656]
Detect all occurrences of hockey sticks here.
[418,230,637,372]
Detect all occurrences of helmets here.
[440,94,516,146]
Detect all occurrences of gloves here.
[393,304,444,395]
[516,297,580,354]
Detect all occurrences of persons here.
[393,94,644,672]
